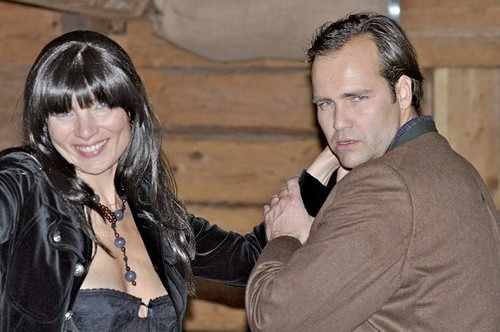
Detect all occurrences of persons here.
[245,11,500,332]
[0,29,342,332]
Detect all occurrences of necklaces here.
[90,194,136,286]
